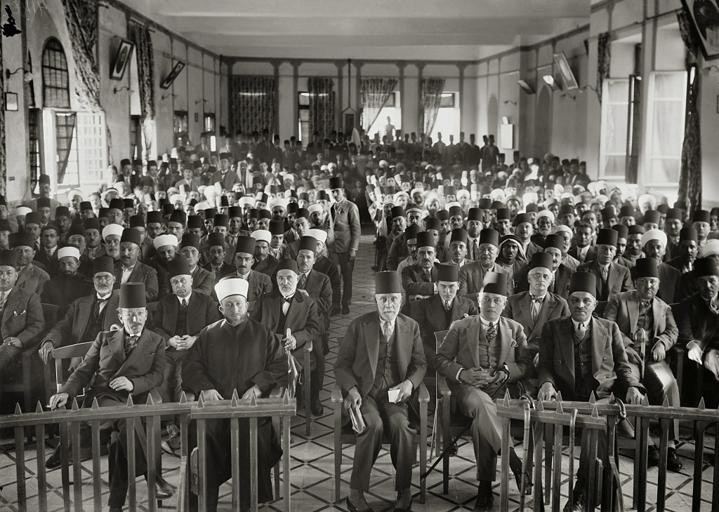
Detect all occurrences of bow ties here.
[123,264,134,272]
[531,294,543,304]
[280,297,293,304]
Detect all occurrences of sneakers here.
[342,304,350,315]
[667,446,683,473]
[346,494,373,511]
[473,486,495,512]
[513,461,533,496]
[393,492,414,511]
[647,444,662,467]
[144,473,178,500]
[45,444,71,470]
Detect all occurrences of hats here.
[390,206,405,219]
[213,278,250,303]
[305,130,426,167]
[133,160,142,167]
[527,252,553,274]
[468,207,574,251]
[167,259,191,281]
[79,201,92,213]
[374,271,404,294]
[93,256,114,277]
[55,206,70,219]
[147,161,157,168]
[427,131,494,143]
[513,151,520,159]
[368,167,662,212]
[277,258,299,275]
[38,197,52,208]
[160,162,168,167]
[118,282,147,309]
[437,263,460,283]
[101,223,328,256]
[120,159,131,168]
[596,224,668,249]
[39,174,50,185]
[600,205,683,223]
[694,257,719,279]
[480,272,509,299]
[97,174,344,226]
[497,153,505,162]
[169,131,304,168]
[7,232,35,250]
[405,203,467,249]
[691,207,719,225]
[1,218,12,232]
[0,249,17,267]
[635,257,658,279]
[569,271,597,298]
[57,246,80,261]
[680,227,697,242]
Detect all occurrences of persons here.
[0,129,718,511]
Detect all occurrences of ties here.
[443,300,451,312]
[94,298,105,318]
[485,322,497,343]
[383,321,393,341]
[601,267,607,283]
[425,269,431,281]
[576,323,586,341]
[300,273,307,288]
[0,292,6,311]
[125,335,138,355]
[181,299,189,311]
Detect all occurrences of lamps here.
[558,93,577,100]
[6,68,33,83]
[161,92,181,102]
[578,84,597,97]
[503,100,518,106]
[114,87,136,95]
[195,97,208,105]
[699,65,719,76]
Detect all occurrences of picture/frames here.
[110,40,134,81]
[160,61,185,89]
[554,51,579,91]
[516,80,536,95]
[682,0,719,60]
